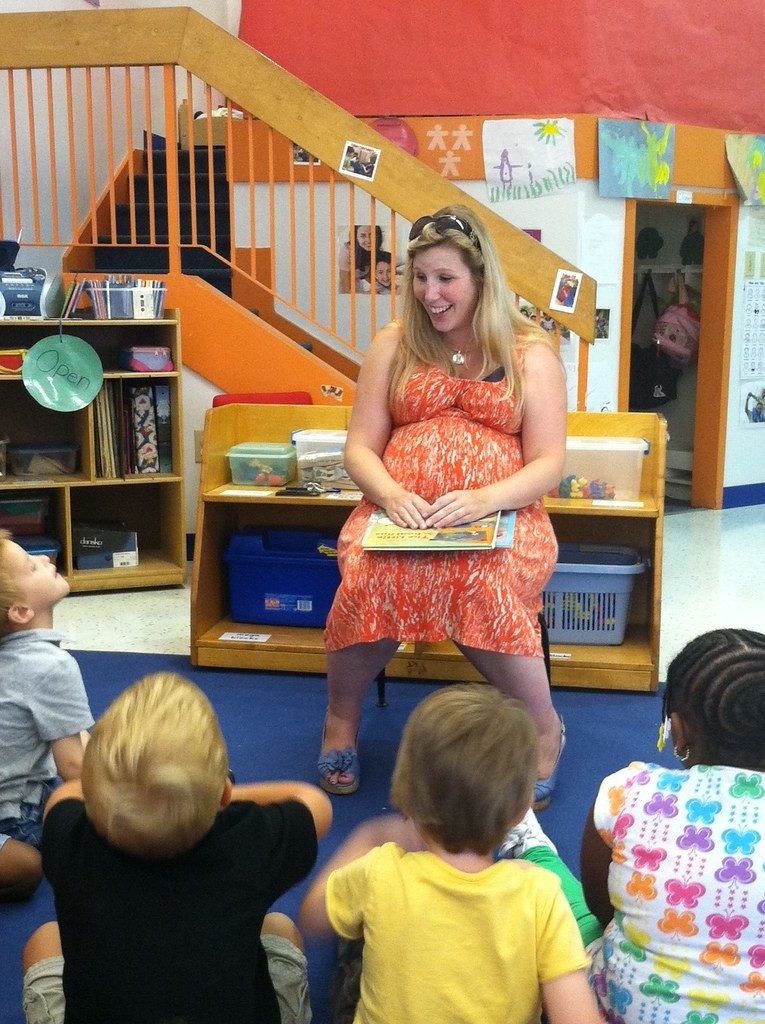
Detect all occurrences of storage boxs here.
[547,435,649,502]
[228,531,343,628]
[0,339,173,572]
[86,287,168,320]
[225,443,295,485]
[292,428,366,491]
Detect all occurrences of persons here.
[343,146,377,177]
[21,672,333,1024]
[0,529,95,906]
[338,225,401,294]
[596,311,607,338]
[492,628,765,1024]
[563,325,570,340]
[556,273,578,308]
[316,202,567,812]
[745,389,765,423]
[297,680,605,1024]
[519,308,555,334]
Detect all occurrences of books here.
[91,377,173,479]
[59,274,166,319]
[361,505,516,551]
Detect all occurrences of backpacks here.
[652,271,701,367]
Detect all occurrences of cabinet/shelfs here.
[190,404,668,693]
[0,309,187,594]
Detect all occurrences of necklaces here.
[441,339,482,365]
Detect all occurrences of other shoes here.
[318,706,361,795]
[531,714,566,812]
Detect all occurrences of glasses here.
[409,214,480,248]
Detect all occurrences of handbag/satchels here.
[629,343,684,409]
[680,220,706,265]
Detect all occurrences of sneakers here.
[497,807,559,859]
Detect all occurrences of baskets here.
[539,543,651,645]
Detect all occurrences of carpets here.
[0,648,765,1024]
